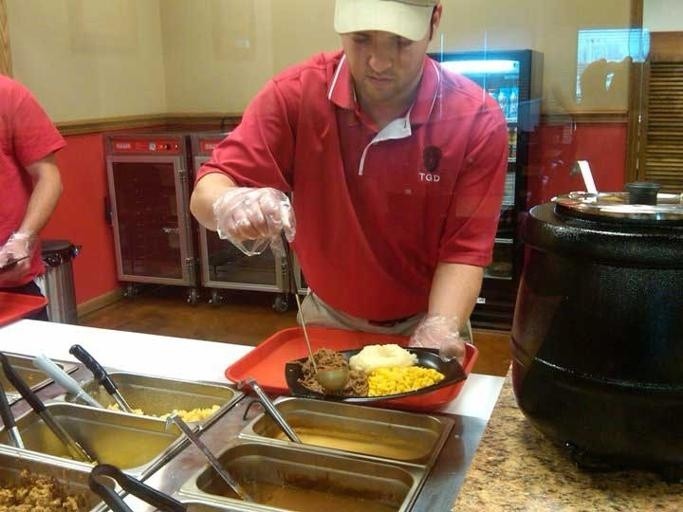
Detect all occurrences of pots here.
[509,182,682,485]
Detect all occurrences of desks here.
[0,319,683,512]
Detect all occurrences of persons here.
[0,70,66,322]
[191,0,509,364]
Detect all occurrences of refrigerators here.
[425,49,544,334]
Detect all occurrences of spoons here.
[267,212,350,390]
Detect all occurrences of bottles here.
[512,128,518,160]
[489,89,495,101]
[510,87,518,120]
[507,128,512,161]
[498,88,508,118]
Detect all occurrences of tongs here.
[87,463,190,511]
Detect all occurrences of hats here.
[334,0,439,41]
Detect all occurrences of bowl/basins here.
[50,369,245,426]
[1,349,76,398]
[235,394,479,468]
[175,437,427,512]
[0,447,122,511]
[0,397,198,476]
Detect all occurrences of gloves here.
[0,230,37,279]
[213,187,296,257]
[408,314,465,365]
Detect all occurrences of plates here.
[283,347,468,412]
[0,291,48,327]
[0,258,48,291]
[224,325,480,414]
